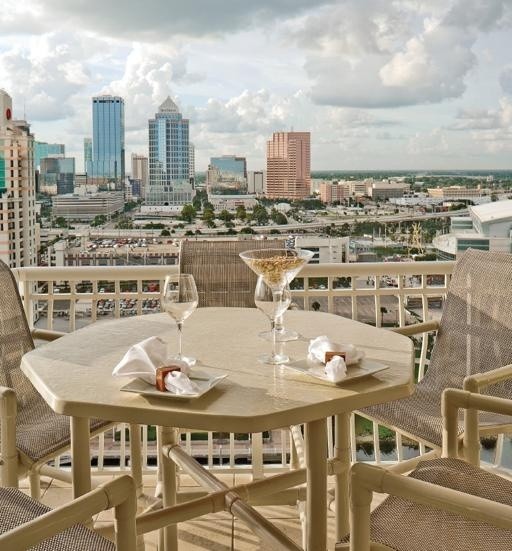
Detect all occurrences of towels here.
[113,337,200,398]
[308,335,364,382]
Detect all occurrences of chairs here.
[0,260,144,488]
[180,238,286,306]
[349,246,512,465]
[333,389,512,551]
[2,474,139,551]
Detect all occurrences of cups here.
[254,272,291,364]
[239,248,315,343]
[161,274,198,366]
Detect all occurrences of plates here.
[285,353,391,385]
[120,364,229,402]
